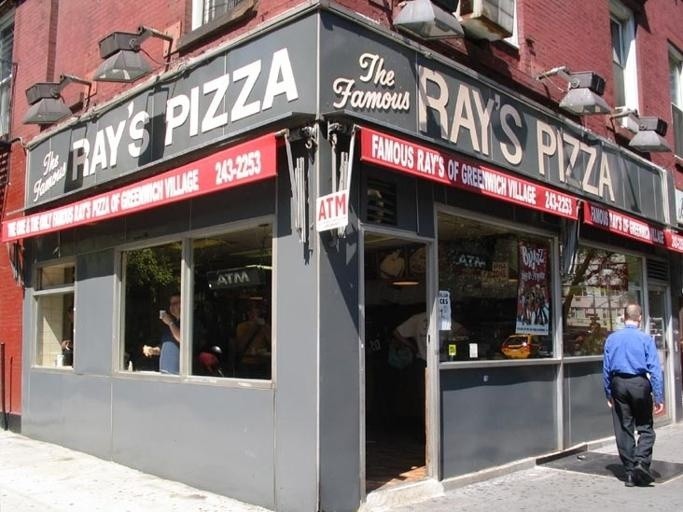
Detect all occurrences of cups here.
[159,310,166,319]
[56,354,63,366]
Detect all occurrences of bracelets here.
[168,321,174,326]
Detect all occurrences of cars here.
[500,334,552,359]
[562,328,615,355]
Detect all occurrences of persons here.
[518,280,549,324]
[143,291,180,374]
[493,349,505,360]
[394,311,467,374]
[235,305,270,364]
[61,305,73,366]
[603,303,665,487]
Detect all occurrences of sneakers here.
[623,470,650,486]
[632,461,655,483]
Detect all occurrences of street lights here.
[604,274,613,331]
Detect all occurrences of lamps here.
[21,72,93,128]
[93,25,173,85]
[605,107,671,154]
[387,0,464,41]
[538,63,613,121]
